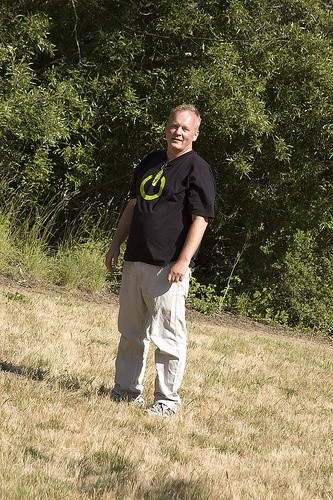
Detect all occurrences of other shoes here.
[104,387,143,409]
[144,401,182,420]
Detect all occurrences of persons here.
[104,103,215,421]
